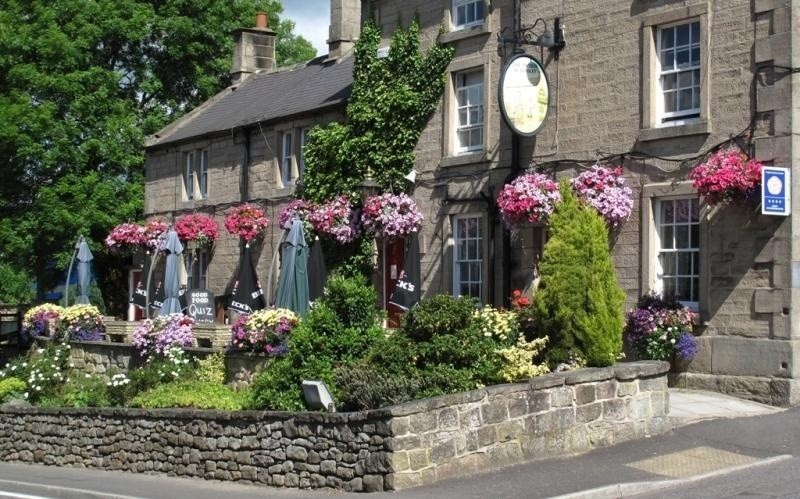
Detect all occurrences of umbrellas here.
[217,237,268,314]
[145,223,184,320]
[267,208,313,317]
[309,236,327,302]
[65,235,94,306]
[151,252,189,313]
[130,251,156,310]
[388,226,421,313]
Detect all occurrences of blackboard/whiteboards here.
[185,287,216,323]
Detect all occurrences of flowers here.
[568,164,634,231]
[510,289,530,312]
[105,223,141,247]
[307,194,356,245]
[495,329,549,381]
[474,305,514,342]
[140,219,170,250]
[277,196,308,228]
[58,305,107,342]
[360,190,425,243]
[222,203,268,249]
[497,171,564,229]
[230,307,300,358]
[622,290,699,368]
[690,143,761,211]
[175,211,218,248]
[131,314,194,365]
[20,303,64,339]
